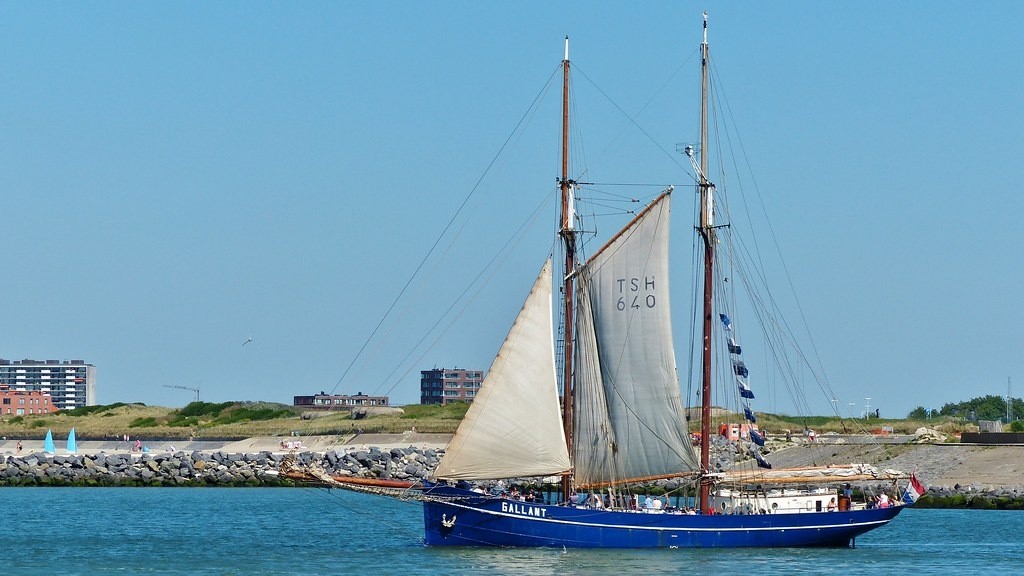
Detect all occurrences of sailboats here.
[276,11,930,549]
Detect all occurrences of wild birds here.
[242,328,252,347]
[724,278,728,282]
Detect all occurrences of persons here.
[843,484,853,511]
[567,489,670,511]
[827,497,837,512]
[480,486,487,494]
[868,490,901,509]
[510,488,545,504]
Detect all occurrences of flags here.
[748,426,765,446]
[718,313,732,331]
[755,449,772,469]
[732,358,748,378]
[902,473,926,506]
[726,336,742,355]
[737,380,755,399]
[742,403,757,423]
